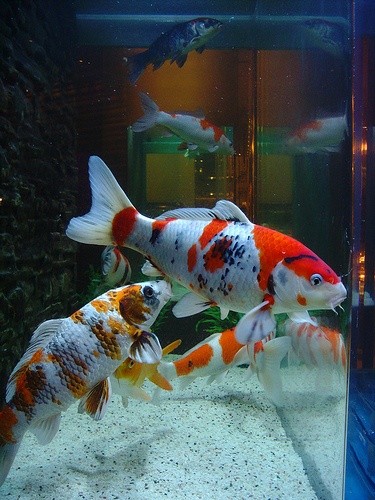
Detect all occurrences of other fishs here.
[244,330,292,408]
[126,17,225,85]
[132,90,237,157]
[284,316,346,381]
[286,114,349,155]
[303,17,344,58]
[152,325,275,405]
[0,280,173,487]
[114,339,182,407]
[65,157,347,346]
